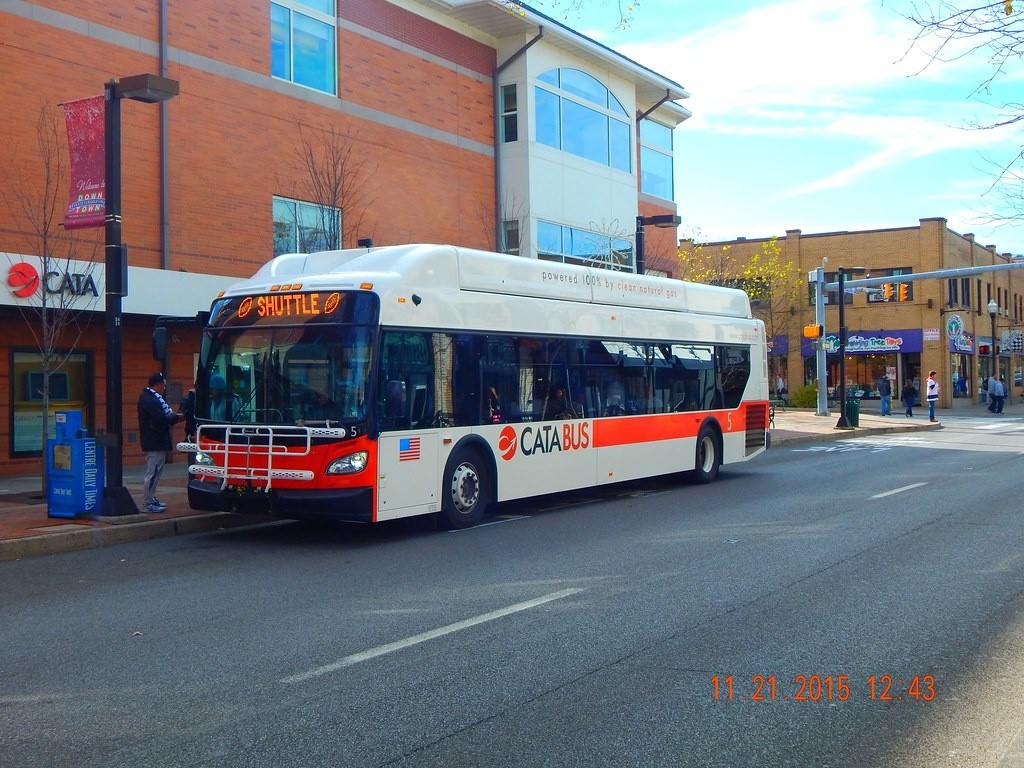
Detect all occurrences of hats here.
[209,375,225,391]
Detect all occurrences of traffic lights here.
[815,325,824,338]
[883,283,894,298]
[899,284,908,302]
[980,346,989,354]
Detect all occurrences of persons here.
[983,373,1006,414]
[901,380,918,417]
[777,374,784,395]
[345,369,393,425]
[878,376,891,416]
[211,375,242,421]
[178,385,195,481]
[553,388,567,419]
[138,372,185,512]
[953,371,968,396]
[487,387,500,422]
[927,371,940,422]
[636,388,663,413]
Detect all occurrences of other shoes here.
[905,413,909,417]
[931,419,938,422]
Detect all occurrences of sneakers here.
[150,499,168,508]
[141,504,167,512]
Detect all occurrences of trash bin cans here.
[842,398,859,427]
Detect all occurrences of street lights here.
[635,214,681,274]
[100,73,181,516]
[837,265,865,427]
[988,298,998,372]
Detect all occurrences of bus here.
[151,242,771,528]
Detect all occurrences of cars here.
[1014,373,1023,387]
[951,373,959,383]
